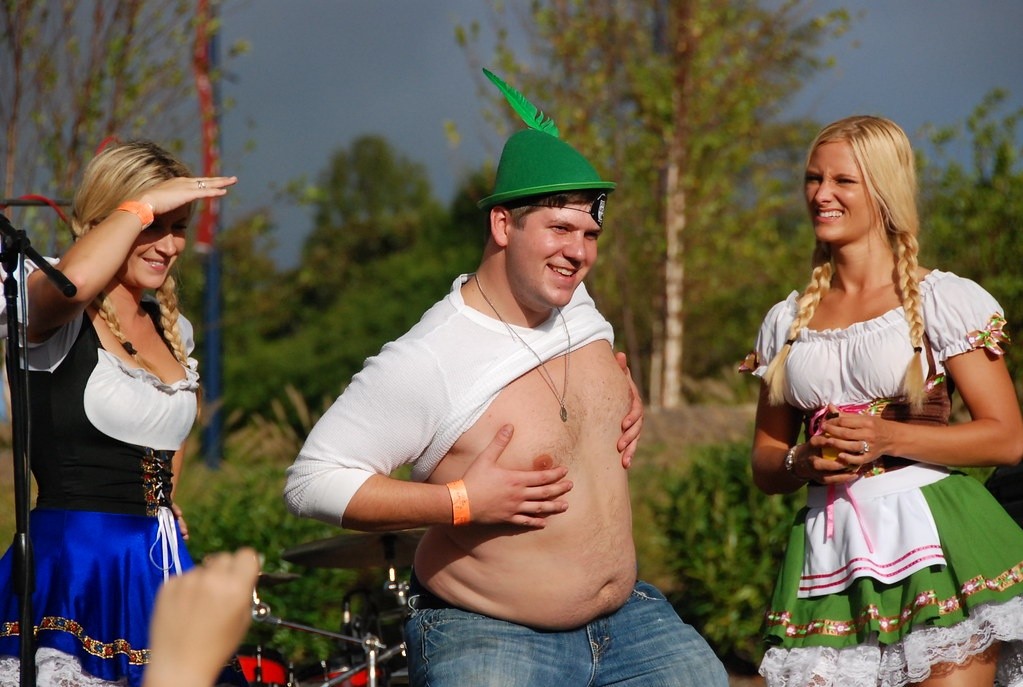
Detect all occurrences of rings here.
[198,181,206,189]
[860,442,869,454]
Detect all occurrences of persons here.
[735,117,1023,687]
[284,128,728,686]
[143,546,259,687]
[0,140,247,687]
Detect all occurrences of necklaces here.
[475,273,571,422]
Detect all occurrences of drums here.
[323,662,388,687]
[234,643,297,687]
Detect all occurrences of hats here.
[476,68,617,209]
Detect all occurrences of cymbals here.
[254,570,300,586]
[280,529,423,568]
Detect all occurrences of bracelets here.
[446,479,471,525]
[116,201,154,231]
[786,443,812,482]
